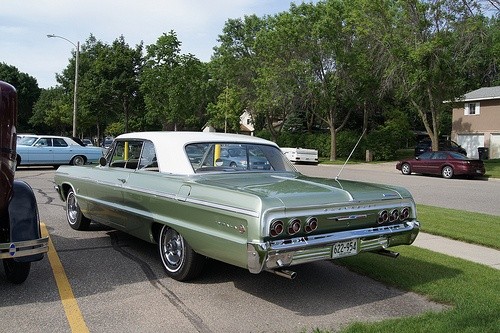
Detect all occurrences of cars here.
[207,146,271,172]
[395,151,486,179]
[101,140,114,148]
[81,139,93,146]
[55,131,421,283]
[414,137,468,157]
[15,134,105,169]
[185,145,205,162]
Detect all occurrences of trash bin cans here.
[478,147,488,160]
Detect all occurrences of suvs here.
[0,79,49,282]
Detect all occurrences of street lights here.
[46,34,79,138]
[207,78,229,133]
